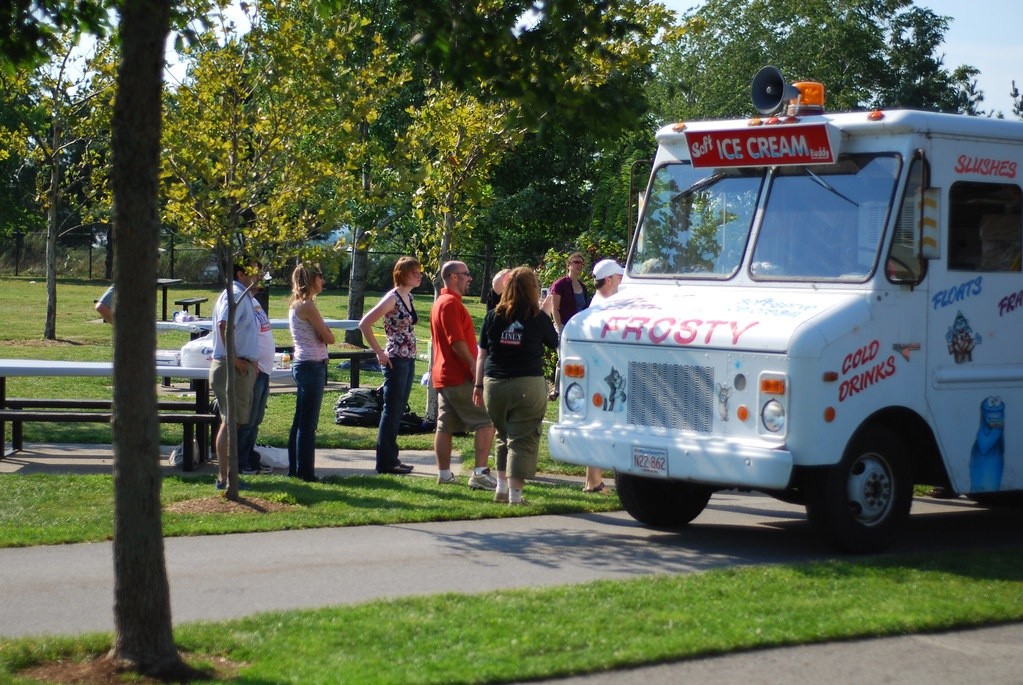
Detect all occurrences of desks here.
[157,279,183,321]
[0,357,293,470]
[157,317,365,389]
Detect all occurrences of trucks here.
[549,110,1023,545]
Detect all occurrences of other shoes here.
[493,493,534,508]
[215,479,252,491]
[583,482,606,494]
[546,389,560,401]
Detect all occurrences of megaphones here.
[749,65,799,114]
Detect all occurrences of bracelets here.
[474,384,483,389]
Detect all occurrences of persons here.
[584,259,629,495]
[286,261,336,483]
[93,246,167,328]
[430,261,498,490]
[471,266,561,508]
[356,252,423,473]
[204,253,282,491]
[484,269,512,314]
[546,253,591,402]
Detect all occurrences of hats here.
[591,260,625,281]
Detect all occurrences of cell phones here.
[541,288,549,300]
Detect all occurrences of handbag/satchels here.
[181,330,214,367]
[168,438,201,468]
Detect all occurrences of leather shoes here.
[378,463,415,474]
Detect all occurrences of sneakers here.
[467,468,497,490]
[436,473,459,485]
[242,462,274,475]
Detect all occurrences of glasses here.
[407,271,424,279]
[316,272,323,279]
[457,271,471,278]
[572,260,584,265]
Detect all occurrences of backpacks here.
[336,387,436,433]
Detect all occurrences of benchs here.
[175,297,208,316]
[0,407,218,465]
[320,348,384,389]
[6,395,216,451]
[274,346,296,360]
